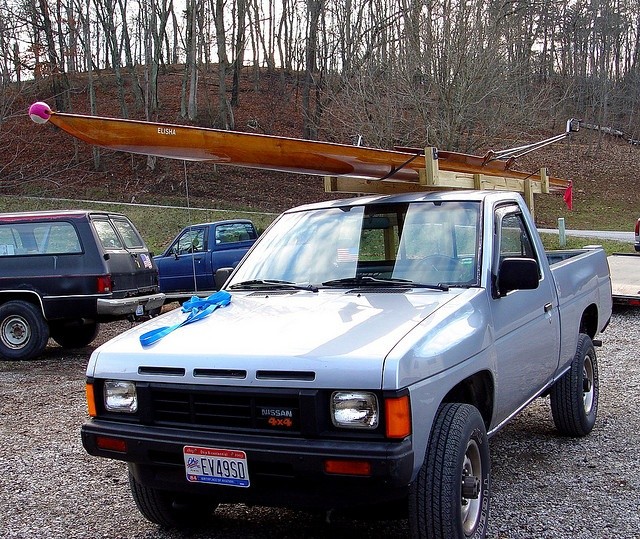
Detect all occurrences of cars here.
[633,218,640,252]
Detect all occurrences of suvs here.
[0,210,166,362]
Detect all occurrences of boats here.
[30,100,581,215]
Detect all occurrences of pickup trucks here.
[151,219,259,307]
[83,189,614,539]
